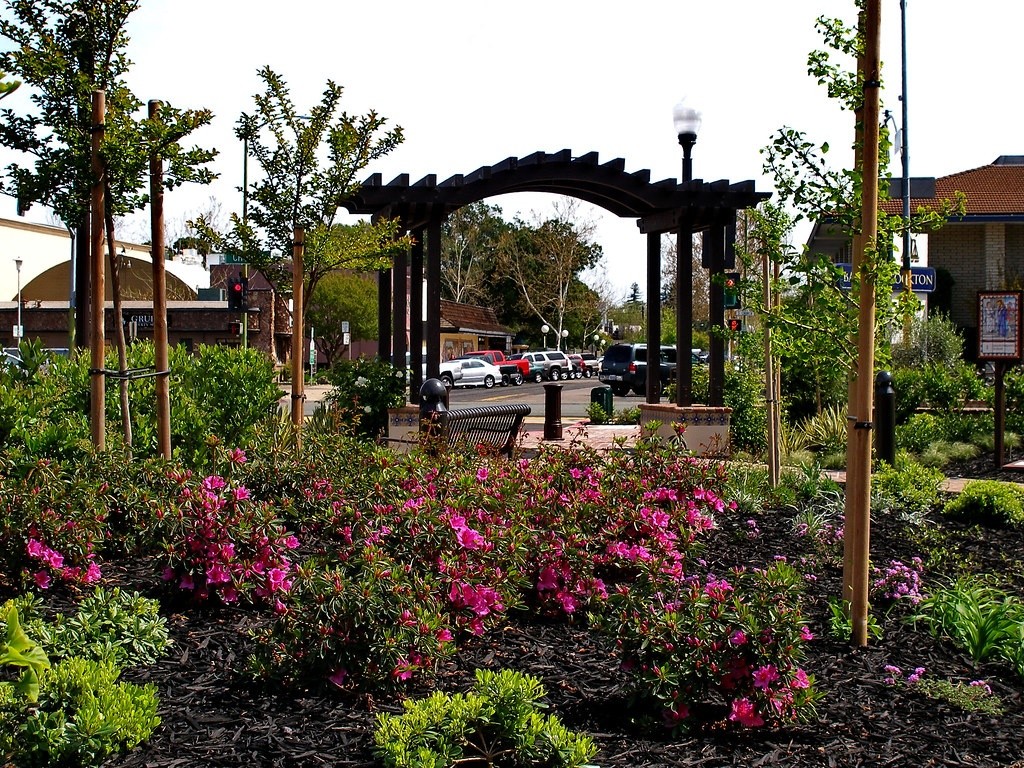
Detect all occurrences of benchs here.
[378,404,531,458]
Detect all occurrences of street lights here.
[671,95,702,407]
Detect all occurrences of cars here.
[673,344,745,374]
[443,358,502,390]
[0,346,71,378]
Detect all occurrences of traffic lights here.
[728,318,742,332]
[725,272,742,311]
[15,256,22,348]
[228,277,242,312]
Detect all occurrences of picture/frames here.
[972,290,1024,362]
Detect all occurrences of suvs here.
[597,344,705,397]
[506,349,605,383]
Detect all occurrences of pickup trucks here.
[390,351,463,393]
[454,354,519,387]
[465,350,531,386]
